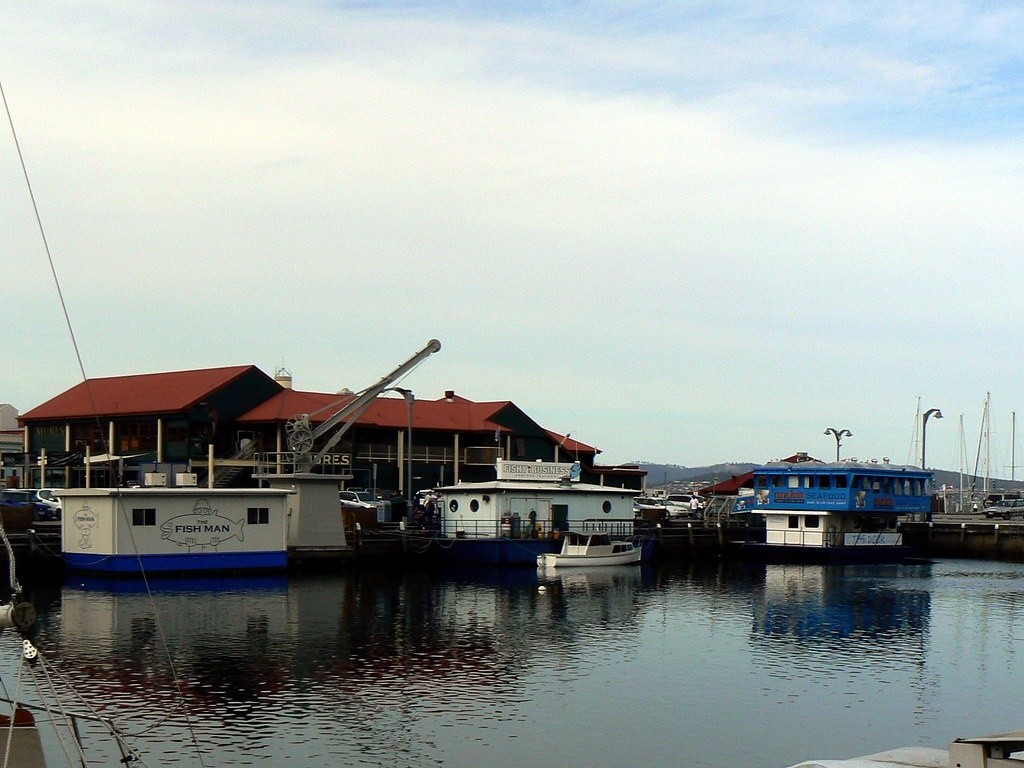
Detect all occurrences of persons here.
[528,508,536,525]
[690,495,698,520]
[389,489,424,524]
[701,498,708,519]
[423,491,438,522]
[240,436,252,454]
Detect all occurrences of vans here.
[984,498,1024,520]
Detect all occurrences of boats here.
[537,564,642,581]
[537,531,643,568]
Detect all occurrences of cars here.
[0,480,711,532]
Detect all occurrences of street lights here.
[823,427,853,463]
[922,409,943,471]
